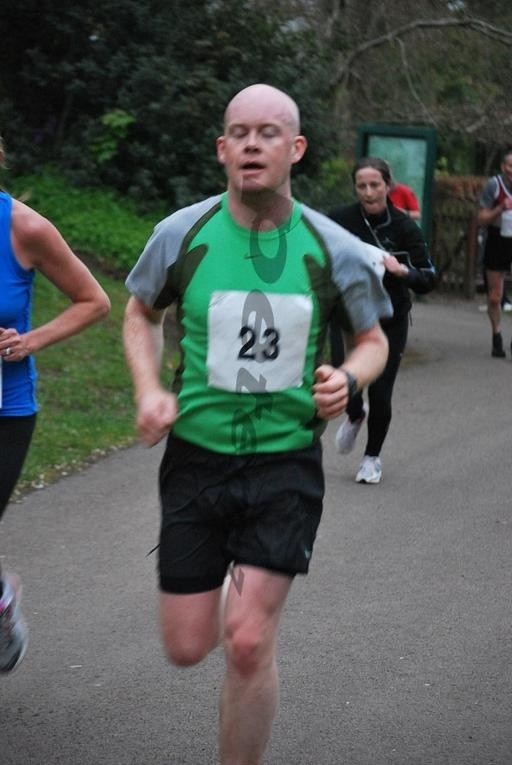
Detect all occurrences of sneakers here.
[0,574,27,673]
[491,333,505,356]
[335,402,368,454]
[356,454,382,484]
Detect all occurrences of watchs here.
[337,367,359,402]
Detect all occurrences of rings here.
[6,347,12,355]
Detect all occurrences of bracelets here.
[404,209,410,218]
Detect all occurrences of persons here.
[382,159,423,221]
[0,190,113,679]
[121,81,395,764]
[325,155,441,488]
[475,150,512,360]
